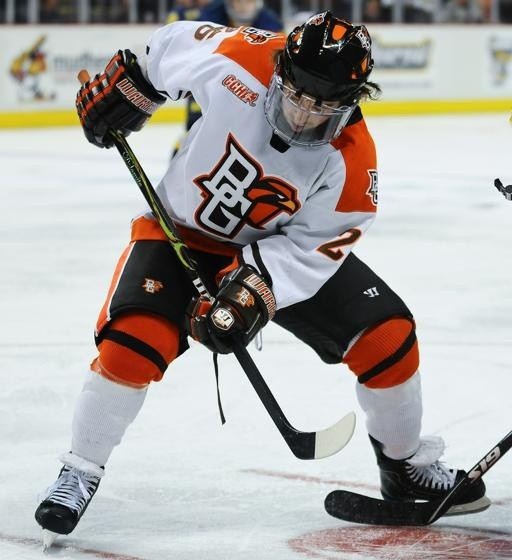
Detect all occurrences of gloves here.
[74,48,168,150]
[181,265,278,356]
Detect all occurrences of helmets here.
[262,9,375,152]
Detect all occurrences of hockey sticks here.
[326,430,511,526]
[78,69,355,458]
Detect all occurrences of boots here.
[31,449,106,538]
[366,432,487,508]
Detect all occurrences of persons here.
[188,0,285,131]
[34,18,485,534]
